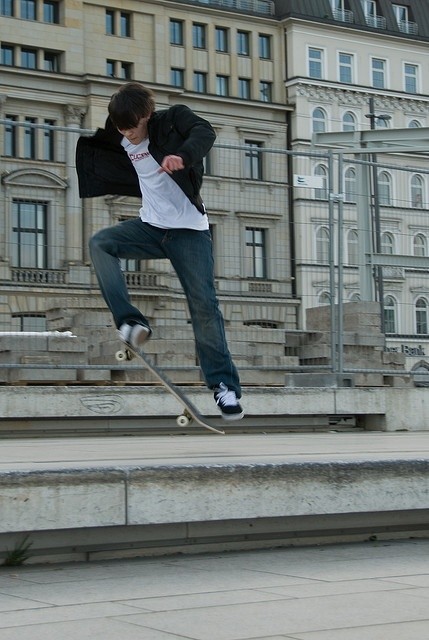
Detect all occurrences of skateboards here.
[114,338,225,434]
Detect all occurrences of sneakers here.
[119,324,152,350]
[214,382,245,422]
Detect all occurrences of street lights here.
[364,96,393,335]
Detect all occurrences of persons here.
[78,87,245,421]
